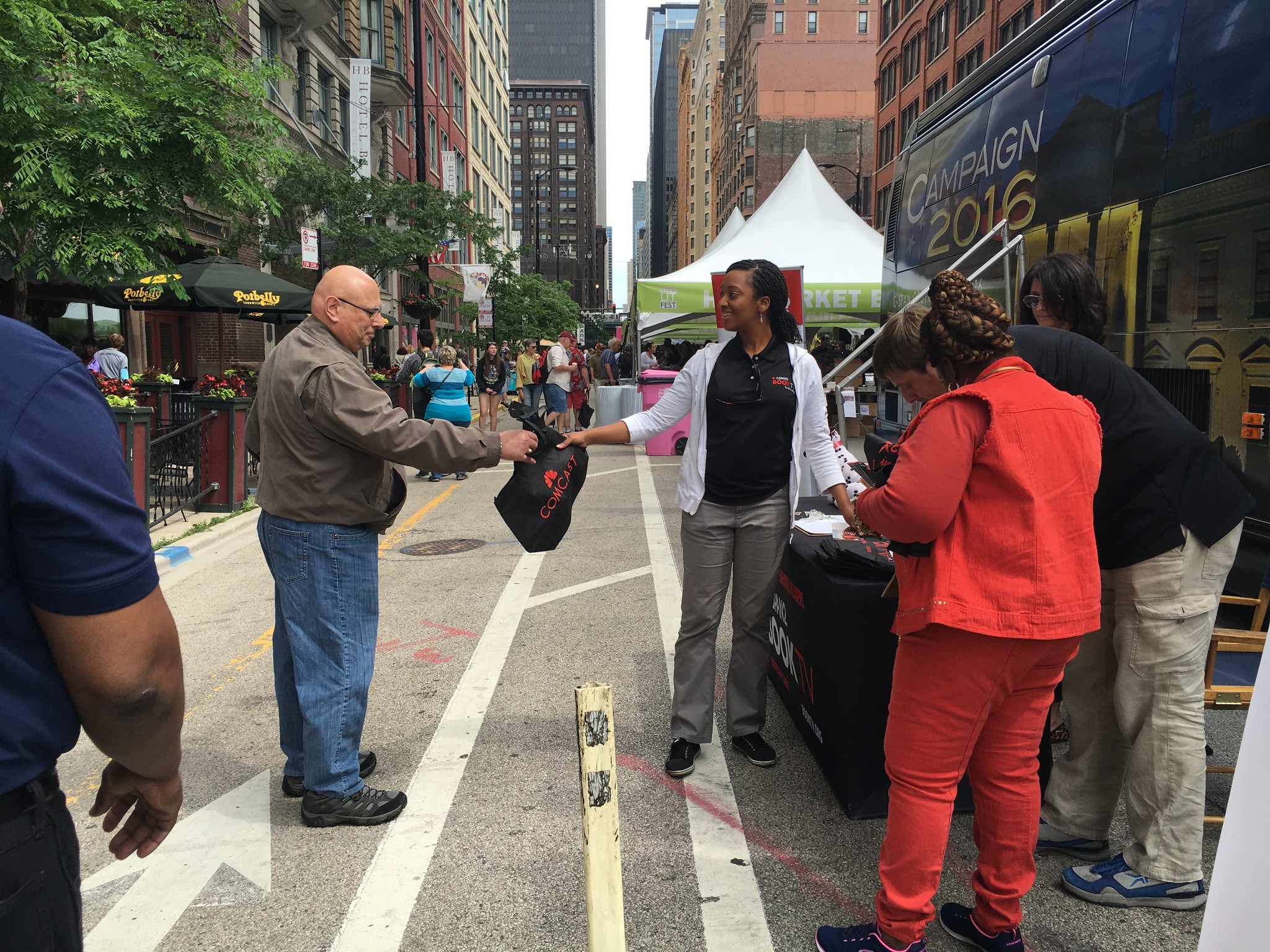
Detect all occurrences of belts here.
[601,377,616,379]
[1,772,58,815]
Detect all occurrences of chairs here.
[150,417,198,526]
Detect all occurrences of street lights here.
[535,165,580,353]
[817,117,863,216]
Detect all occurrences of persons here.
[413,345,475,482]
[372,330,719,434]
[556,259,863,776]
[810,329,883,414]
[1017,252,1108,743]
[393,329,453,479]
[80,337,100,367]
[88,332,129,381]
[244,265,539,827]
[0,314,188,952]
[814,270,1104,952]
[872,301,1257,911]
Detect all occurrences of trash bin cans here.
[636,368,692,457]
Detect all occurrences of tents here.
[635,148,885,376]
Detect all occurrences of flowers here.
[130,366,173,383]
[367,365,398,381]
[89,368,138,408]
[197,362,258,399]
[388,292,448,313]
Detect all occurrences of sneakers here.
[415,471,469,482]
[301,790,407,828]
[281,750,376,798]
[500,402,510,408]
[548,423,584,434]
[665,732,1210,952]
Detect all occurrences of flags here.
[459,266,491,302]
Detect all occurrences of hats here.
[558,331,576,342]
[821,334,832,345]
[596,343,633,350]
[502,340,508,343]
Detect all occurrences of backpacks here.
[417,350,440,372]
[532,345,564,384]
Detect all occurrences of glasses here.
[487,342,496,344]
[834,346,840,348]
[338,298,382,319]
[528,346,538,348]
[715,360,762,405]
[1022,293,1067,308]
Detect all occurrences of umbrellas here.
[106,247,315,376]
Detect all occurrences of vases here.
[405,304,441,320]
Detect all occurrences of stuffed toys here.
[802,426,867,508]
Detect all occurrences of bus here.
[871,0,1268,700]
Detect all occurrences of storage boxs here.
[830,401,877,437]
[825,358,863,388]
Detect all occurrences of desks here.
[764,495,1053,822]
[825,391,877,436]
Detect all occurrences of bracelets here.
[500,442,502,459]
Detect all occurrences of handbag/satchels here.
[863,432,931,558]
[86,350,101,372]
[495,401,590,553]
[578,388,594,428]
[422,392,432,408]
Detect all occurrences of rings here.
[527,448,533,455]
